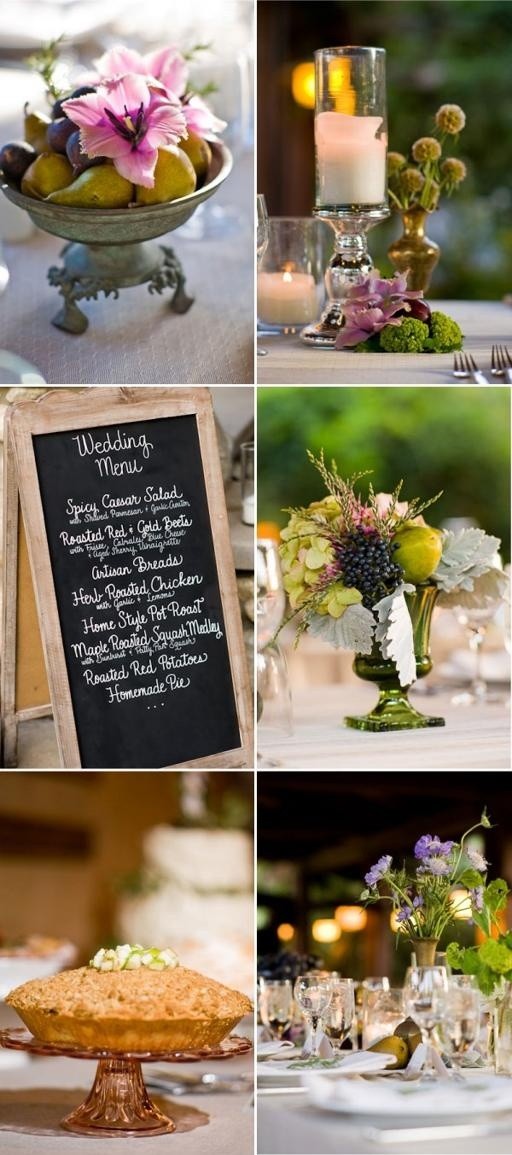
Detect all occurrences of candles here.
[314,111,387,209]
[258,272,315,326]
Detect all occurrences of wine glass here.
[454,571,500,710]
[258,970,480,1089]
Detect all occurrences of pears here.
[1,95,217,210]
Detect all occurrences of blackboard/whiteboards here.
[7,386,253,772]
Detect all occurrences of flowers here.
[27,33,232,191]
[269,443,502,688]
[330,264,426,352]
[350,808,512,996]
[384,102,467,211]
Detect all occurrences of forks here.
[491,345,512,382]
[453,352,488,384]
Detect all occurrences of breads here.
[5,943,252,1048]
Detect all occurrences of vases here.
[341,582,446,731]
[392,937,442,1054]
[387,206,442,296]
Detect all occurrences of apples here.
[386,521,444,585]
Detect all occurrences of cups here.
[256,540,285,654]
[257,216,324,331]
[311,46,390,205]
